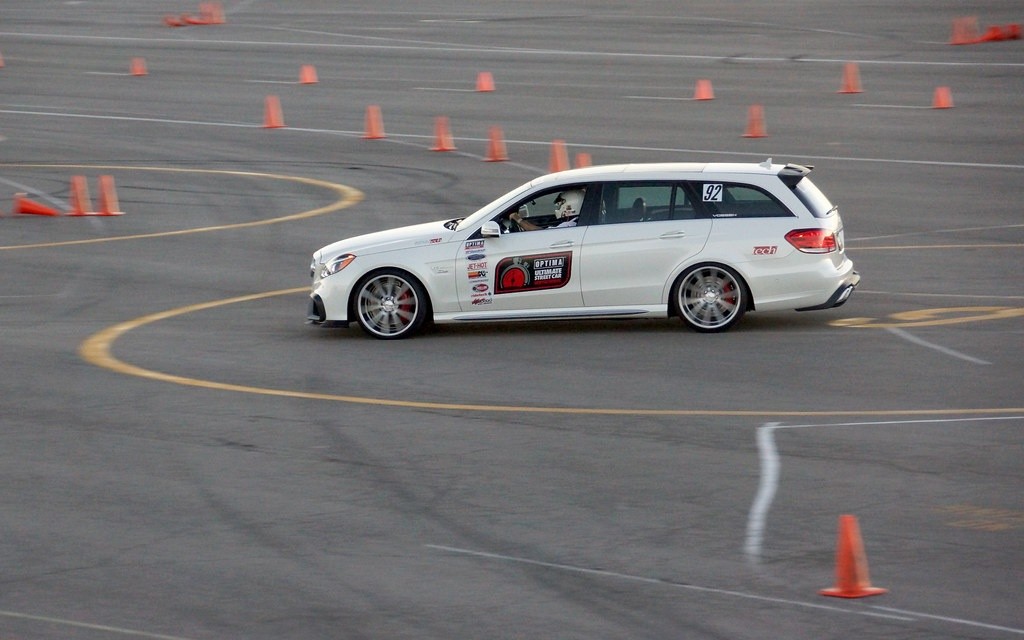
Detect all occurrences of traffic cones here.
[360,105,385,138]
[836,62,864,94]
[261,95,288,127]
[482,125,511,162]
[979,25,1004,40]
[129,58,149,76]
[65,175,125,216]
[695,80,715,100]
[548,140,591,173]
[11,192,59,215]
[932,87,955,108]
[741,105,768,138]
[429,116,456,150]
[477,72,496,91]
[819,514,888,597]
[299,65,320,83]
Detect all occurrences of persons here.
[509,189,585,232]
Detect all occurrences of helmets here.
[554,188,586,220]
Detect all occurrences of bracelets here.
[517,219,522,223]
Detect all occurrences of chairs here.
[631,196,648,221]
[601,199,607,224]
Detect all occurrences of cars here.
[303,157,861,340]
